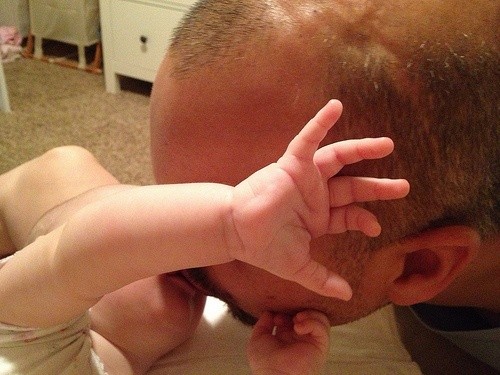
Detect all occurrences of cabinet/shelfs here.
[97,1,203,97]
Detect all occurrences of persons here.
[150,0,500,332]
[0,99,411,375]
[0,145,419,374]
